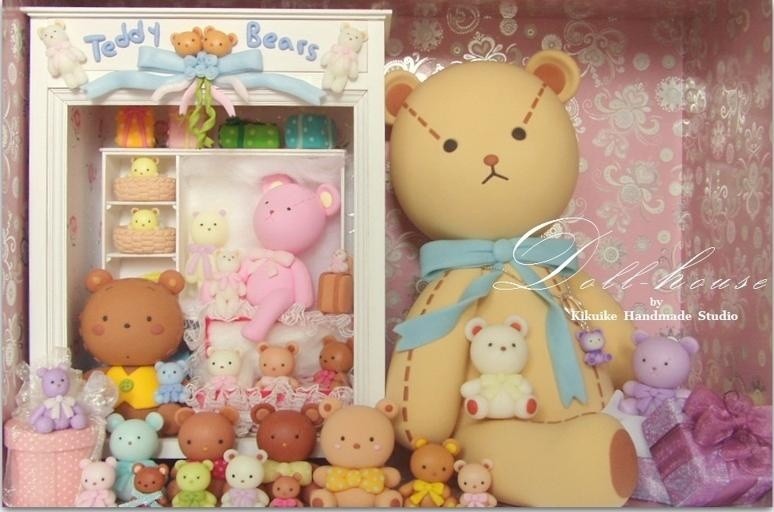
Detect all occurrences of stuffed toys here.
[28,8,700,509]
[26,6,699,512]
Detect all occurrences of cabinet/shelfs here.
[94,142,347,291]
